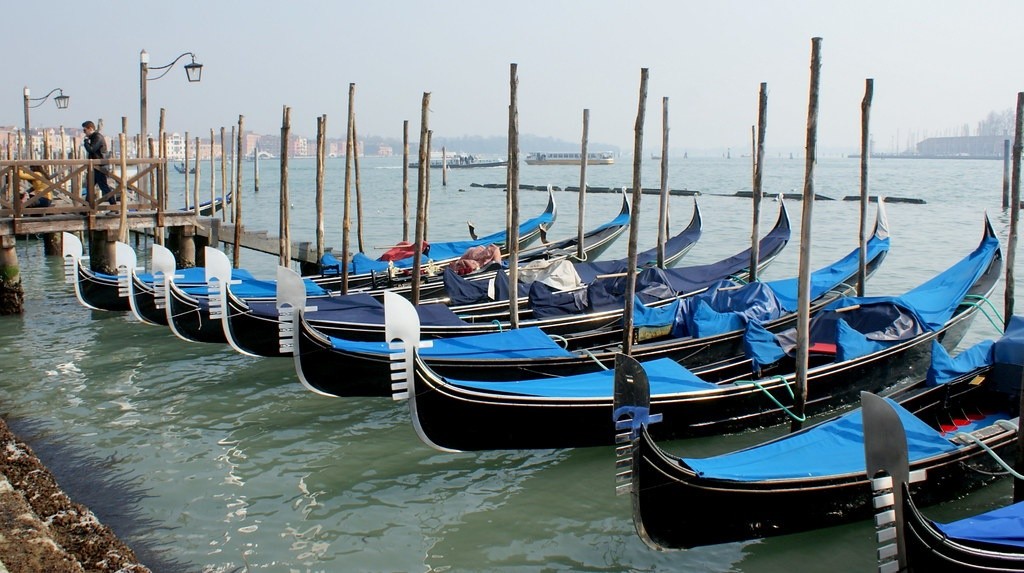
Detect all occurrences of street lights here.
[140,49,202,211]
[24,86,69,159]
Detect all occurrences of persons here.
[80,121,118,216]
[19,165,52,217]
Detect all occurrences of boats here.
[276,195,889,401]
[61,179,701,346]
[173,163,196,175]
[409,152,509,169]
[858,389,1024,573]
[611,313,1024,553]
[385,212,1003,452]
[524,150,613,166]
[206,186,792,359]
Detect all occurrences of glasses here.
[84,127,89,132]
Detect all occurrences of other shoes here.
[106,211,119,215]
[80,212,89,216]
